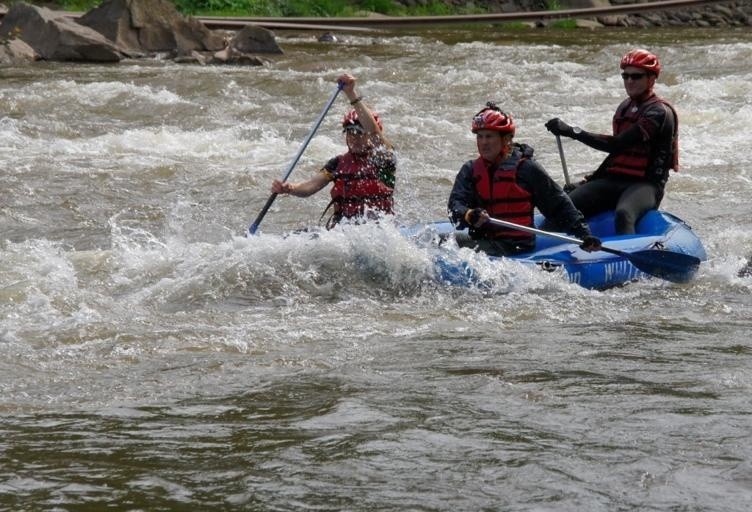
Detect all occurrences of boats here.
[283,210,708,297]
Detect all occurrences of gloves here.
[545,118,573,137]
[464,207,491,228]
[579,235,602,253]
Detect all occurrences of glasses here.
[620,72,648,81]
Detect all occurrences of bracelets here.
[350,95,364,106]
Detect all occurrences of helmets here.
[342,110,382,133]
[471,107,515,136]
[619,49,660,77]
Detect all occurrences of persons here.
[271,79,397,241]
[448,107,600,257]
[543,47,678,235]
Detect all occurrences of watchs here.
[572,126,582,140]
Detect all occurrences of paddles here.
[483,210,700,284]
